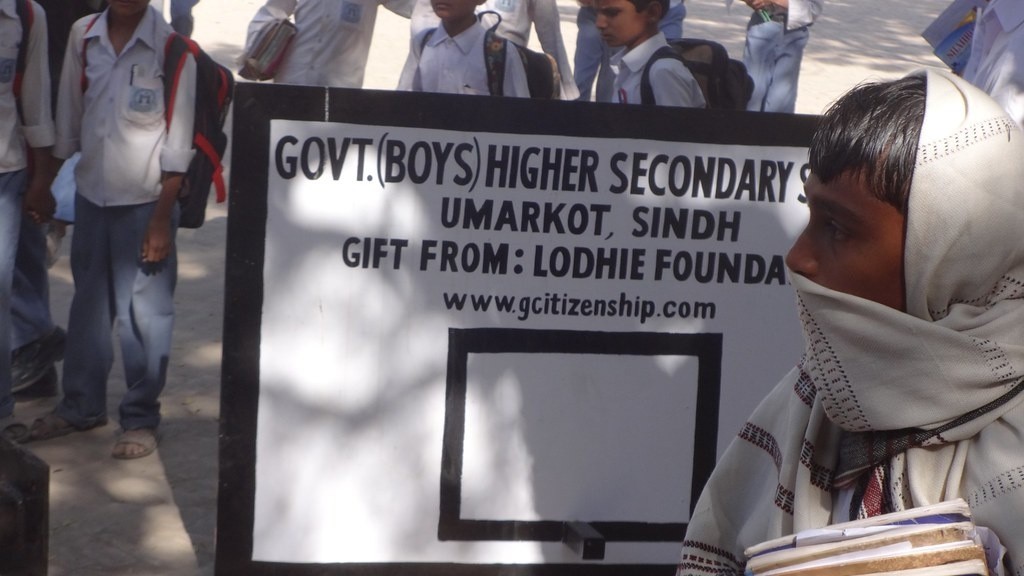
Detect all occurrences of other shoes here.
[1,417,29,444]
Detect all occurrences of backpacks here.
[640,37,754,109]
[81,13,234,229]
[419,10,561,101]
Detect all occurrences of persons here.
[399,0,821,115]
[962,0,1024,128]
[677,66,1024,576]
[0,0,195,461]
[239,0,418,89]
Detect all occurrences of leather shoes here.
[10,326,69,392]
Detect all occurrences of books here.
[921,0,982,75]
[239,20,296,81]
[746,498,1007,576]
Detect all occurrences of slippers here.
[112,426,162,460]
[29,413,76,439]
